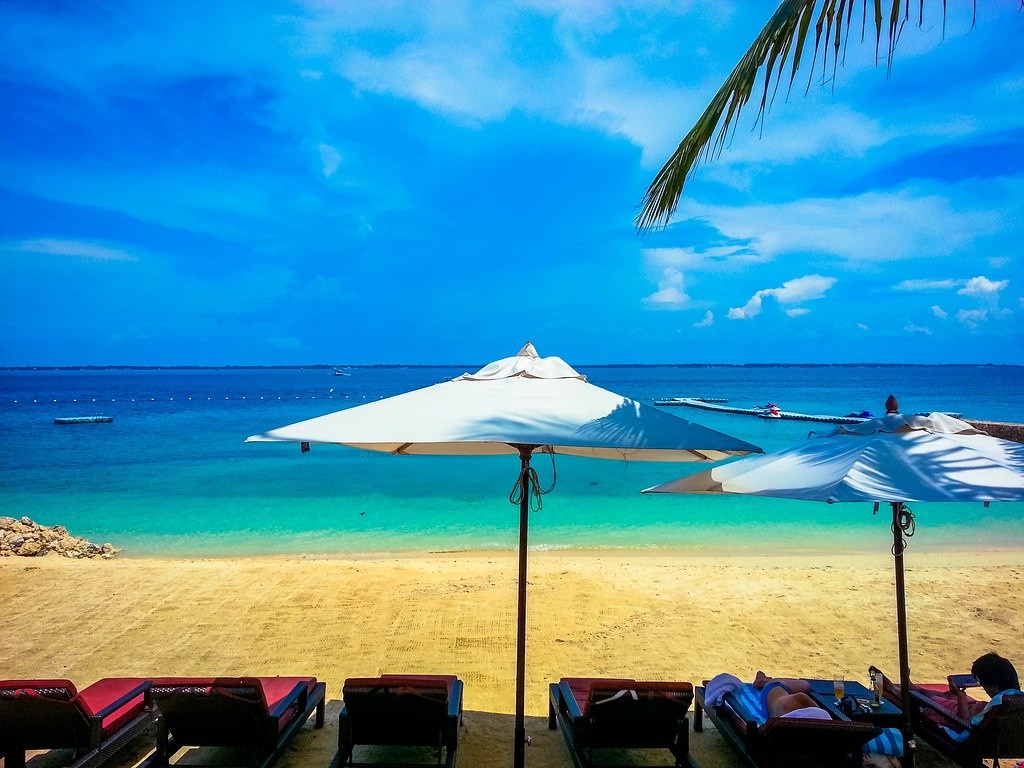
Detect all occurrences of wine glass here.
[833,674,844,706]
[874,673,885,705]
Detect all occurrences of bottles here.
[868,671,880,707]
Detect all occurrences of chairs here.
[693,683,882,768]
[0,678,162,768]
[329,674,463,768]
[136,677,326,768]
[548,678,701,768]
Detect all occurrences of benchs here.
[883,684,1024,768]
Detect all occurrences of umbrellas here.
[640,394,1024,768]
[242,341,765,768]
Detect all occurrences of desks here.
[799,678,909,768]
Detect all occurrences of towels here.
[704,673,744,707]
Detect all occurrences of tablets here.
[951,674,981,687]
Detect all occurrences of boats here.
[334,370,343,376]
[757,409,782,419]
[844,409,875,418]
[750,402,781,411]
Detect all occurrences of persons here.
[753,670,832,720]
[868,653,1024,743]
